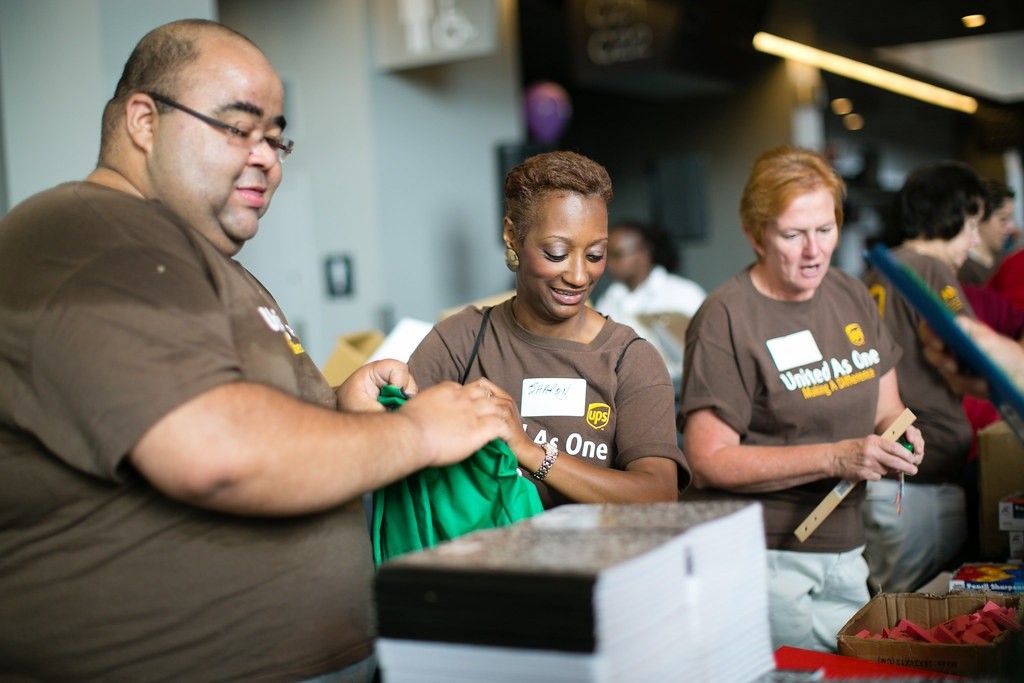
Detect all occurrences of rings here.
[487,389,494,398]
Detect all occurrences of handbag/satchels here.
[372,383,545,578]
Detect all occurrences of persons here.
[407,151,1024,683]
[0,17,514,683]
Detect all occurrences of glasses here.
[145,90,296,164]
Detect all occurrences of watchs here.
[530,442,558,480]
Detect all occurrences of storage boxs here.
[835,590,1024,679]
[948,562,1024,595]
[997,490,1024,532]
[1008,532,1024,559]
